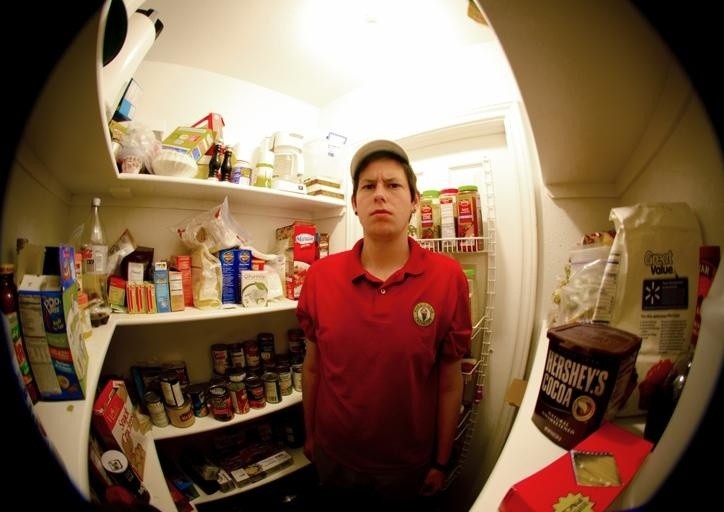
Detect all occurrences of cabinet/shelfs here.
[410,163,497,493]
[468,0,722,512]
[0,1,347,511]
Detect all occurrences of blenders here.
[268,131,306,194]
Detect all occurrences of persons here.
[296,139,471,512]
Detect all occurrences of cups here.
[122,156,142,174]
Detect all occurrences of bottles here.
[463,269,478,334]
[78,197,109,327]
[419,185,483,252]
[152,149,198,178]
[145,390,169,428]
[210,144,273,189]
[100,11,164,124]
[1,238,40,405]
[640,242,723,450]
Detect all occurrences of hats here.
[350,138,409,177]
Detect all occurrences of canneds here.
[144,329,305,428]
[1,263,40,406]
